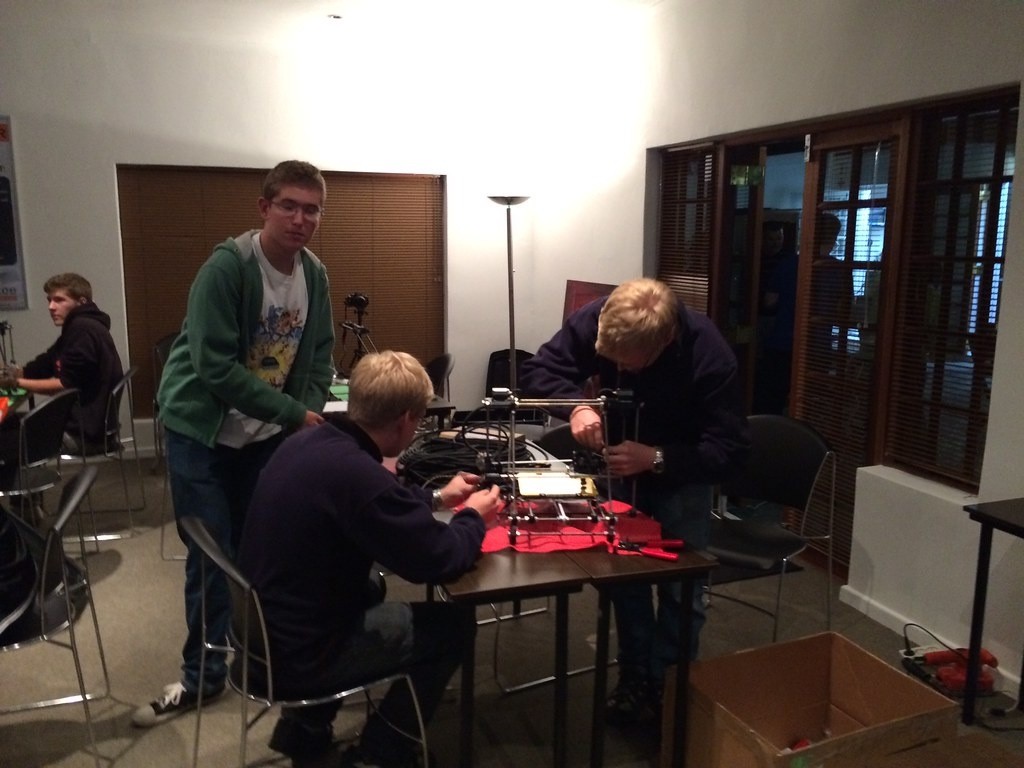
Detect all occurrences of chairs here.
[0,336,839,768]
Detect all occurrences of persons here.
[0,273,124,520]
[518,276,753,745]
[131,162,337,729]
[234,349,502,768]
[737,214,855,414]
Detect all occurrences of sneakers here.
[603,659,683,727]
[131,682,222,729]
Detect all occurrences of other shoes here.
[342,745,436,767]
[267,718,334,755]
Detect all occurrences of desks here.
[555,544,719,768]
[961,500,1024,723]
[412,489,591,768]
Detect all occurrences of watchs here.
[653,444,665,473]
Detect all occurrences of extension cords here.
[898,644,942,663]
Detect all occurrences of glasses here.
[267,196,326,221]
[622,351,658,375]
[411,409,430,433]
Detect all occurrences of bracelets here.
[432,488,442,506]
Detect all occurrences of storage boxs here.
[683,632,1024,768]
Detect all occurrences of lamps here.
[491,194,531,397]
